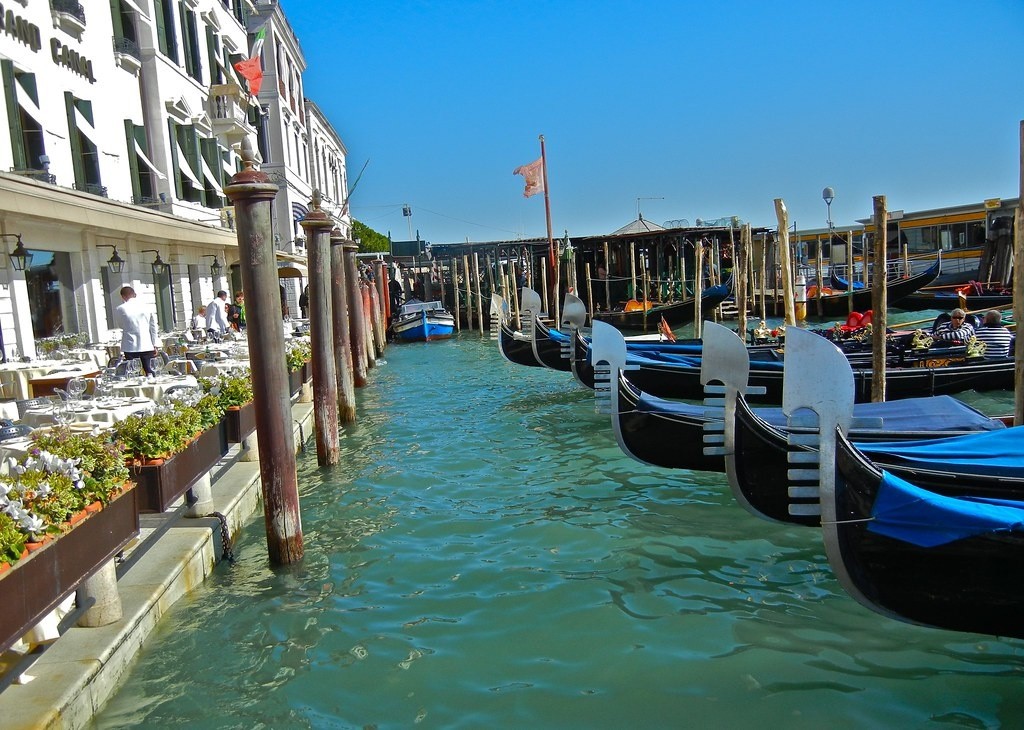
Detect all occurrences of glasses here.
[952,316,965,320]
[982,316,988,321]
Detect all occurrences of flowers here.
[0,426,128,566]
[196,364,253,410]
[284,330,310,376]
[111,387,225,459]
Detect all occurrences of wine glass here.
[126,360,135,382]
[95,374,108,403]
[150,358,160,379]
[74,376,87,401]
[63,403,77,434]
[206,332,214,345]
[155,356,165,380]
[52,402,66,429]
[132,358,142,381]
[102,378,113,405]
[37,346,47,363]
[68,379,79,403]
[213,330,220,344]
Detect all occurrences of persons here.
[206,290,231,344]
[115,287,159,377]
[596,260,607,303]
[963,310,1013,358]
[704,257,718,288]
[932,308,974,343]
[194,291,247,332]
[388,277,402,305]
[516,268,526,296]
[357,259,375,281]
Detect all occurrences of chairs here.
[0,424,34,441]
[15,397,53,420]
[170,360,199,375]
[116,361,143,376]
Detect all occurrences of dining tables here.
[0,322,313,474]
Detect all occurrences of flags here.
[234,25,266,96]
[514,157,546,198]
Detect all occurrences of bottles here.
[205,346,210,358]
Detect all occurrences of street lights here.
[821,186,837,276]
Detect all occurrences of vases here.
[0,480,140,657]
[303,358,313,383]
[129,417,228,513]
[290,367,304,397]
[224,398,256,444]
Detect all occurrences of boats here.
[745,249,944,317]
[773,323,1024,640]
[588,319,1016,473]
[699,318,1023,527]
[565,286,734,329]
[561,291,1016,401]
[489,292,926,366]
[519,284,977,370]
[828,268,1014,312]
[390,298,457,342]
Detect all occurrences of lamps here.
[202,254,222,275]
[96,245,125,273]
[0,233,33,271]
[142,249,167,275]
